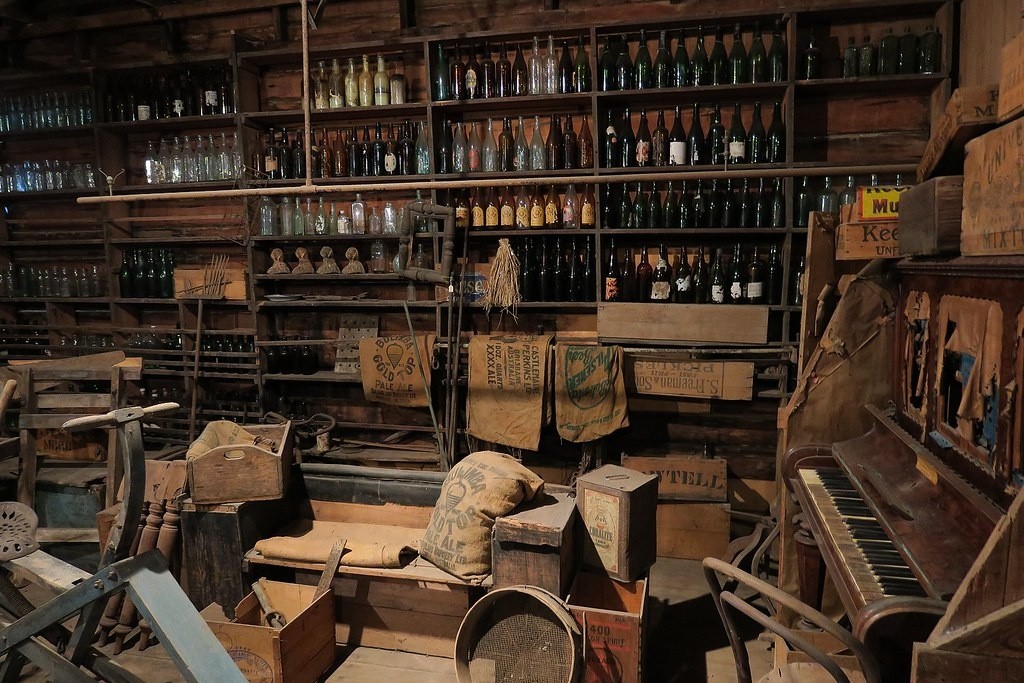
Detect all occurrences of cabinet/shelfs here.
[0,0,954,446]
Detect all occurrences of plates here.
[264,295,304,301]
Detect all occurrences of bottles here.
[803,24,942,80]
[140,387,180,439]
[513,236,595,302]
[60,334,115,356]
[277,396,312,449]
[300,19,788,110]
[268,336,316,375]
[603,237,805,305]
[0,262,19,298]
[252,100,785,178]
[414,244,428,269]
[119,248,174,298]
[214,390,259,423]
[371,238,389,274]
[796,174,903,228]
[129,322,183,370]
[0,86,97,191]
[20,266,102,298]
[105,67,242,184]
[258,176,784,234]
[192,334,256,374]
[406,280,416,302]
[0,318,43,365]
[393,252,399,272]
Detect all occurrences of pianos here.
[781,254,1024,683]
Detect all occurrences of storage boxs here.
[436,263,493,304]
[174,270,251,300]
[97,421,731,683]
[836,202,904,259]
[896,31,1024,255]
